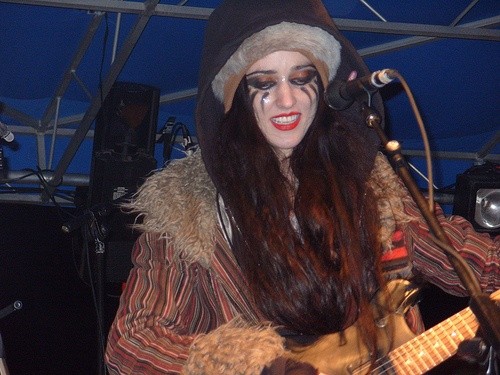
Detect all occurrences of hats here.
[211,22,341,117]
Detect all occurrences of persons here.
[105,0,500,375]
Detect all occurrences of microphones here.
[323,69,393,110]
[181,125,194,156]
[0,122,15,142]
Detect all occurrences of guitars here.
[286,278,500,375]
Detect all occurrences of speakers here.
[86,82,159,219]
